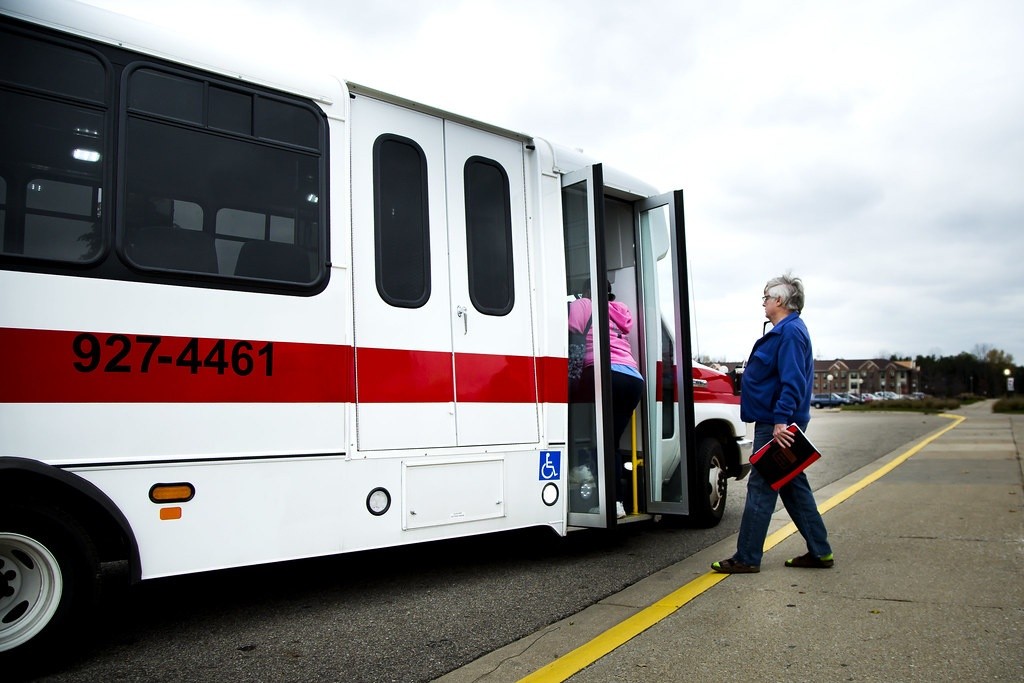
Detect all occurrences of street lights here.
[827,373,834,408]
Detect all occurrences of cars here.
[837,393,864,404]
[854,391,906,401]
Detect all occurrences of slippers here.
[712,559,760,573]
[785,553,834,568]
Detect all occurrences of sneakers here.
[589,501,627,519]
[569,463,596,490]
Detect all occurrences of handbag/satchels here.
[568,301,592,384]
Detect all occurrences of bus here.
[0,1,758,680]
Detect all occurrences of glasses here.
[762,295,770,302]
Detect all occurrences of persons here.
[711,277,834,574]
[568,278,644,519]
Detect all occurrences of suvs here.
[811,393,850,408]
